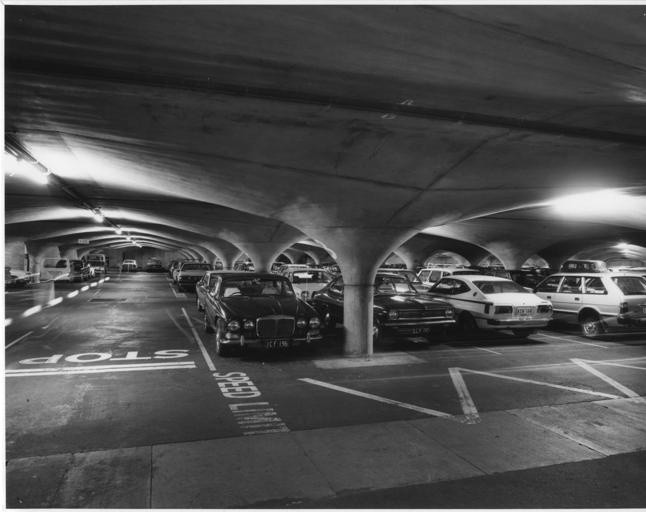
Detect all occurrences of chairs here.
[223,287,240,297]
[261,282,279,296]
[377,283,396,296]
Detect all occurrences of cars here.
[167,258,324,355]
[123,260,137,270]
[147,258,161,271]
[6,253,106,285]
